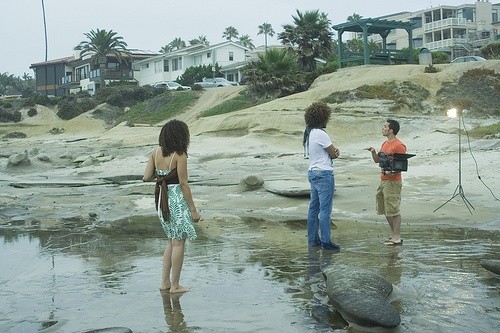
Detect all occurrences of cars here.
[450,55,487,63]
[193,77,240,88]
[153,80,192,91]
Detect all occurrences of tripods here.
[433,108,475,215]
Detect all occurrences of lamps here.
[447,106,461,120]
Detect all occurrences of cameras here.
[378,152,416,171]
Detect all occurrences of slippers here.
[384,237,403,245]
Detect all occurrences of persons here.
[140,119,203,292]
[363,119,408,244]
[302,100,342,251]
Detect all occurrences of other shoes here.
[321,241,339,251]
